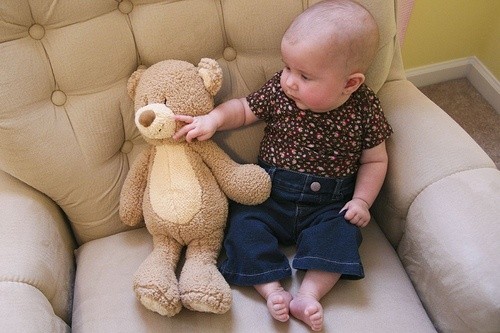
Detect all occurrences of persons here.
[170,1,395,333]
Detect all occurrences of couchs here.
[1,0,500,332]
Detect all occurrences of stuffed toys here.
[116,56,274,320]
